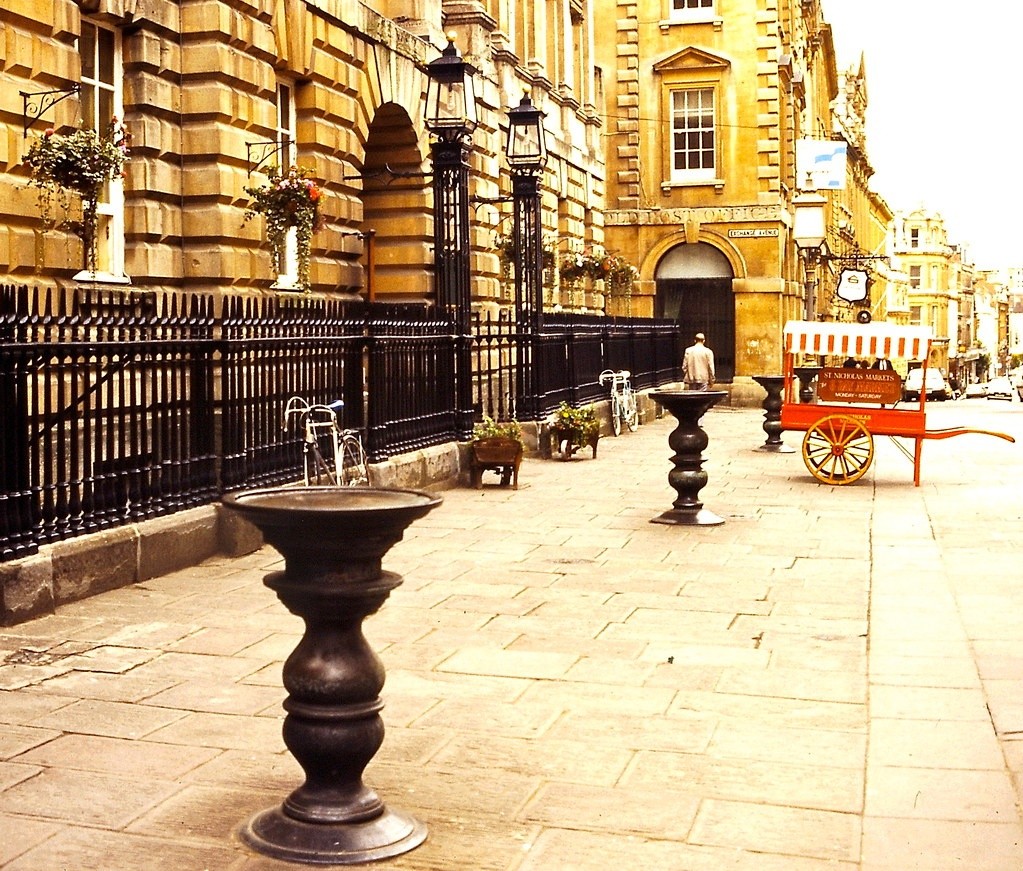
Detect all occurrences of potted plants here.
[468,414,525,491]
[546,399,601,463]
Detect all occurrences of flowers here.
[19,113,134,293]
[240,160,325,295]
[488,220,641,319]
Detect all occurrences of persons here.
[870,355,893,409]
[842,356,862,407]
[680,332,715,392]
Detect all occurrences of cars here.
[939,366,1023,403]
[902,367,946,403]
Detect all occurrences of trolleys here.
[777,318,1016,486]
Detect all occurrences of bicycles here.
[282,396,374,488]
[599,369,639,436]
